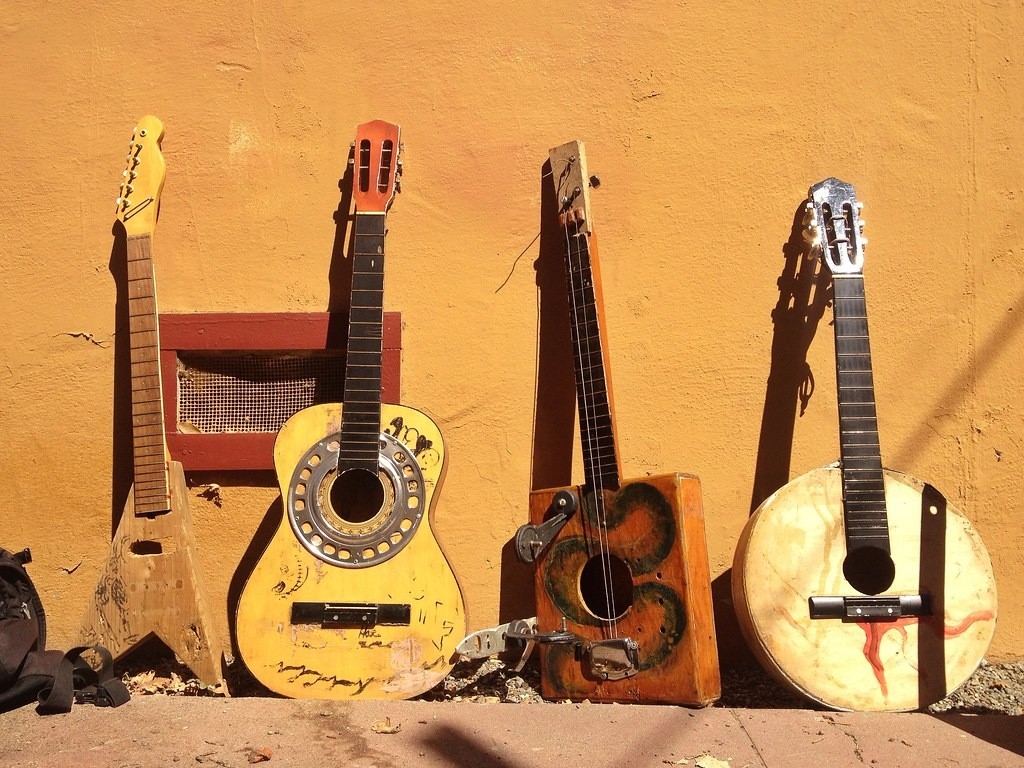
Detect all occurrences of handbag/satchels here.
[1,548,130,714]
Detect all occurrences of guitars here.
[71,117,224,692]
[728,178,999,714]
[524,137,725,710]
[234,117,473,699]
